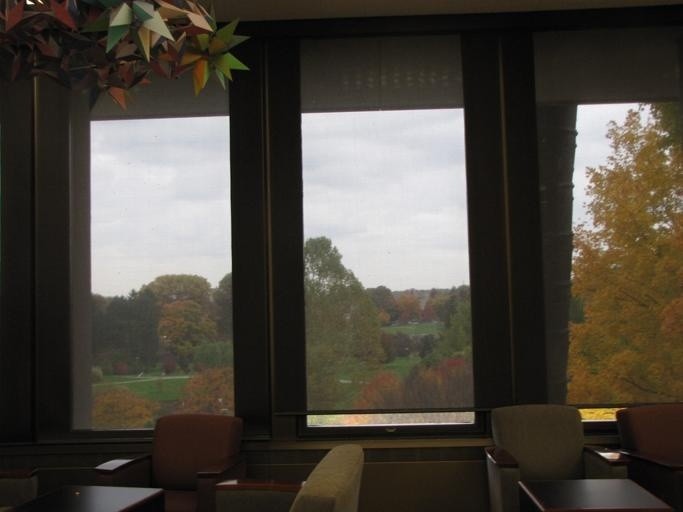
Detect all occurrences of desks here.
[515,475,674,512]
[7,483,169,512]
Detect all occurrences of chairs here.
[481,402,633,511]
[612,402,680,499]
[211,441,366,512]
[93,409,247,512]
